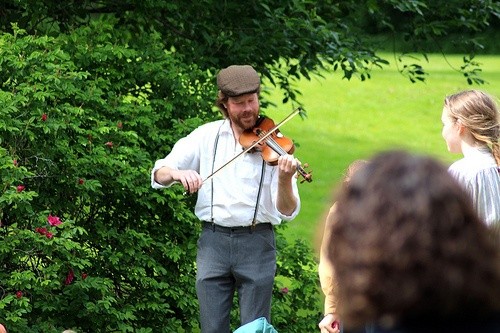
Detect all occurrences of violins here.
[238,116,313,184]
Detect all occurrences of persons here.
[440,90,500,228]
[323,150,500,333]
[318,160,371,333]
[150,65,302,333]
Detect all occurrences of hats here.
[217,64,261,97]
